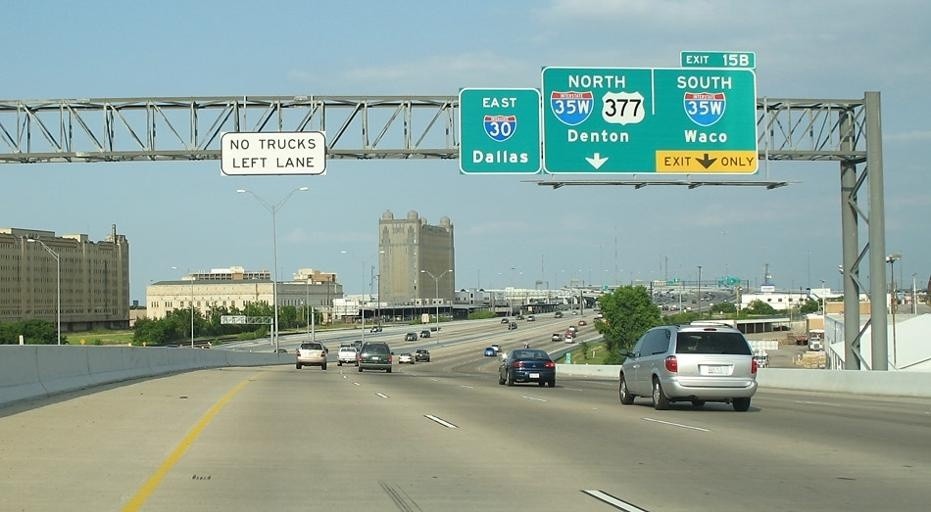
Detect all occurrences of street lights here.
[26,238,61,345]
[421,269,453,344]
[512,266,523,304]
[171,266,194,346]
[885,255,901,364]
[340,248,386,345]
[236,187,308,355]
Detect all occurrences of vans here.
[620,323,757,411]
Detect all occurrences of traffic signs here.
[458,88,540,175]
[540,51,758,175]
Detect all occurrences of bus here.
[752,352,768,368]
[809,329,825,350]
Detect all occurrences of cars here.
[405,330,431,341]
[498,349,556,387]
[415,349,430,362]
[502,314,535,329]
[484,344,500,356]
[552,320,587,343]
[399,353,413,363]
[370,328,381,332]
[295,343,329,370]
[358,342,393,372]
[336,341,361,366]
